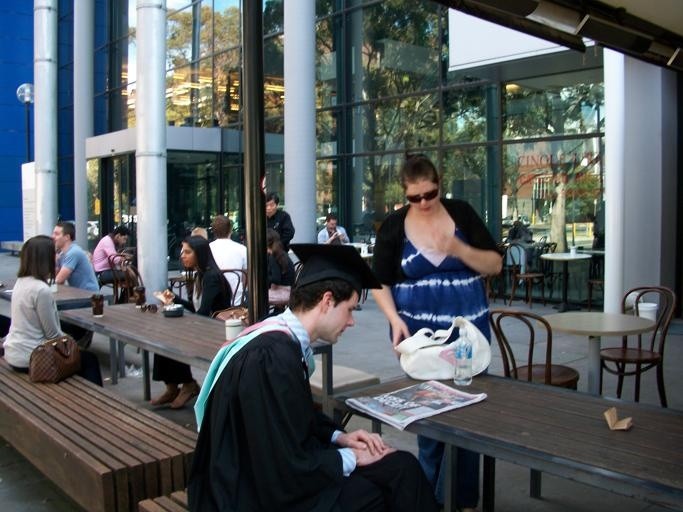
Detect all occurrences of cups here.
[224,319,242,342]
[91,295,102,317]
[133,286,146,308]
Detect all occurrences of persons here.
[314,214,350,244]
[208,215,250,308]
[3,235,104,388]
[93,225,135,305]
[192,228,207,240]
[265,227,296,316]
[49,222,102,350]
[266,190,296,251]
[149,235,233,409]
[191,240,439,511]
[368,152,503,511]
[509,220,532,244]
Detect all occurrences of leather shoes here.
[151,390,177,405]
[171,382,199,408]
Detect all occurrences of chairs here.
[600,286,676,408]
[508,243,546,307]
[489,310,579,391]
[125,265,147,305]
[109,250,137,303]
[588,254,603,312]
[210,269,248,319]
[166,270,193,297]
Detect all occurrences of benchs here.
[0,358,199,512]
[310,358,380,422]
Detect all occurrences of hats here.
[289,244,380,290]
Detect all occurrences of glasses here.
[406,189,438,204]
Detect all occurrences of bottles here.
[453,328,472,386]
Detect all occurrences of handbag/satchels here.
[394,316,492,380]
[212,307,248,325]
[26,336,77,382]
